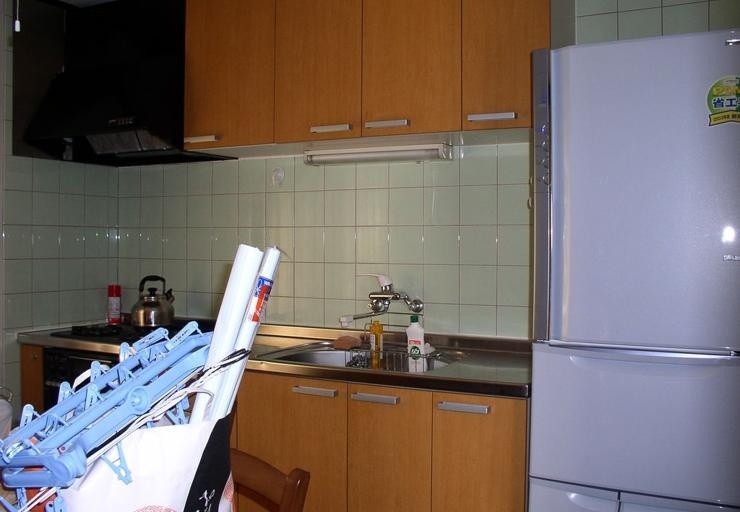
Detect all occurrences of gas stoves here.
[50,318,216,347]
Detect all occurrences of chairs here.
[229,447,311,511]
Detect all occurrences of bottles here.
[405,315,425,354]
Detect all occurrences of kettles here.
[130,275,175,333]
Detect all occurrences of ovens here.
[42,348,148,413]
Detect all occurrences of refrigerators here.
[524,27,740,512]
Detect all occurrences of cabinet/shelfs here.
[273,0,462,155]
[346,383,432,511]
[182,1,277,159]
[462,0,575,147]
[431,390,530,512]
[235,370,346,512]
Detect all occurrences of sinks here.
[257,341,449,372]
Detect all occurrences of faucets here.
[339,300,390,327]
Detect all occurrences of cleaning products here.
[406,315,428,372]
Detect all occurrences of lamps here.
[303,144,452,167]
